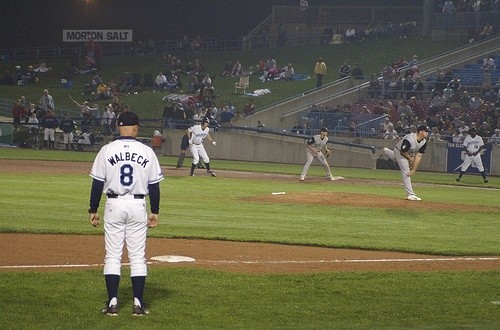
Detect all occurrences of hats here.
[66,113,69,116]
[47,109,51,113]
[467,129,475,134]
[321,127,330,132]
[419,125,432,132]
[203,117,210,123]
[117,111,140,126]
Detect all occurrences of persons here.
[13,0,500,169]
[315,57,327,88]
[300,127,339,181]
[394,125,431,201]
[454,128,488,183]
[88,111,164,317]
[187,117,217,177]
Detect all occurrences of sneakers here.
[484,179,488,183]
[407,195,422,201]
[189,171,194,176]
[207,170,214,173]
[101,304,119,316]
[132,305,149,316]
[456,178,461,181]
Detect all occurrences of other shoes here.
[328,175,335,181]
[300,177,304,181]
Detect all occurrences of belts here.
[108,193,144,199]
[396,146,401,151]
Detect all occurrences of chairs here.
[234,76,250,94]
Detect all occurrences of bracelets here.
[211,141,213,143]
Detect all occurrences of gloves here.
[211,141,217,145]
[188,139,192,144]
